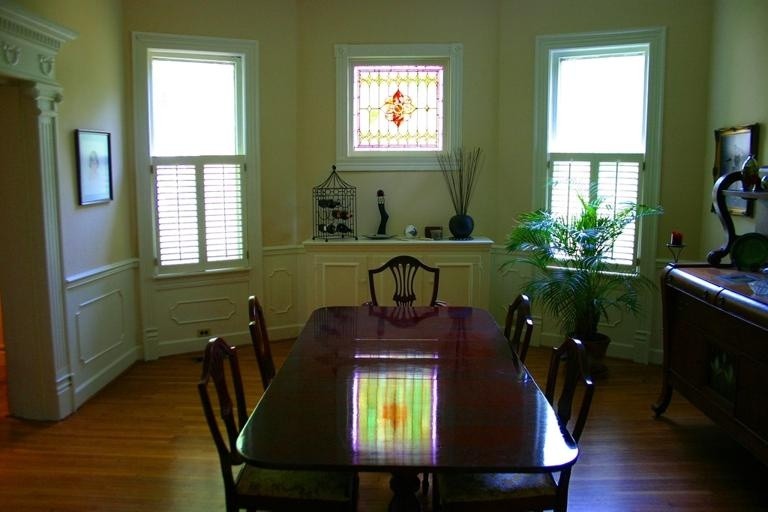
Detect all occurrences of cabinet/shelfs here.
[303,236,495,324]
[651,171,768,467]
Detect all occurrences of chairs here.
[504,294,533,363]
[367,256,440,306]
[197,337,359,512]
[432,338,594,512]
[248,295,276,389]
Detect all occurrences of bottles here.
[740,153,760,192]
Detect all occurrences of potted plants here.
[496,194,664,371]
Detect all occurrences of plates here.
[730,232,768,272]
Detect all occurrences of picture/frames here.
[75,129,113,206]
[711,123,761,216]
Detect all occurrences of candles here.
[671,231,683,244]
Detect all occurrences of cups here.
[430,230,444,242]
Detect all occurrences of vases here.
[449,215,474,238]
